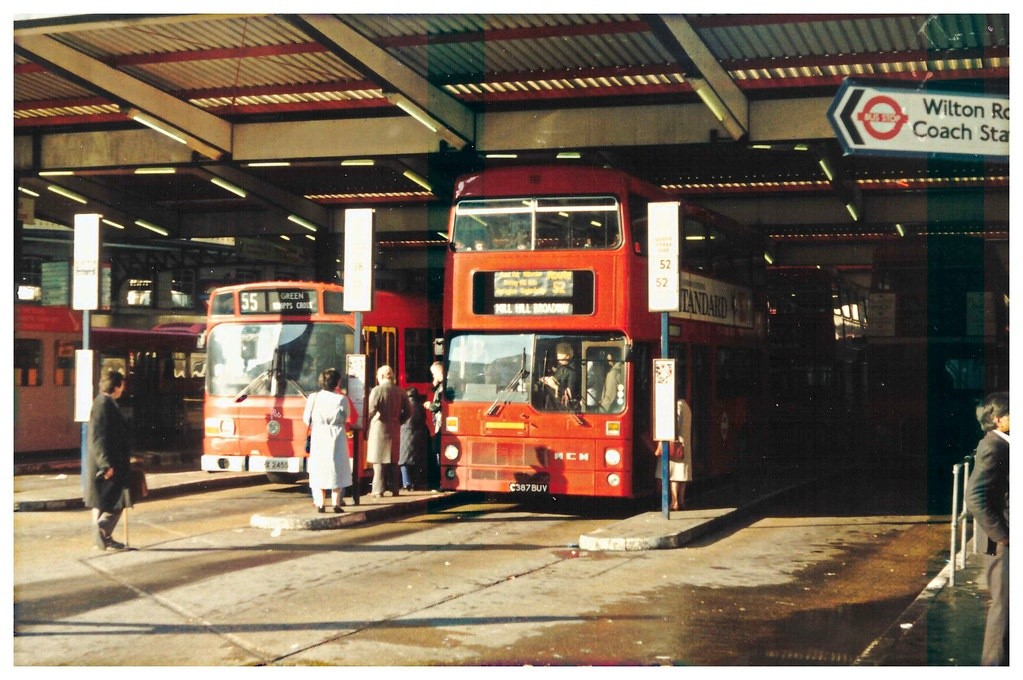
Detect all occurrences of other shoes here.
[318,505,325,512]
[669,505,680,511]
[334,506,344,512]
[403,485,417,491]
[373,494,383,498]
[95,521,108,550]
[392,492,398,496]
[106,537,124,550]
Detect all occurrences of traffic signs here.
[826,76,1009,164]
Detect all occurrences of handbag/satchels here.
[128,469,147,500]
[655,436,684,460]
[306,436,310,453]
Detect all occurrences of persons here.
[367,365,426,497]
[423,361,444,493]
[540,343,624,413]
[85,371,137,549]
[303,368,359,514]
[964,392,1009,666]
[654,386,692,510]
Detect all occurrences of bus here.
[440,168,757,497]
[201,280,438,483]
